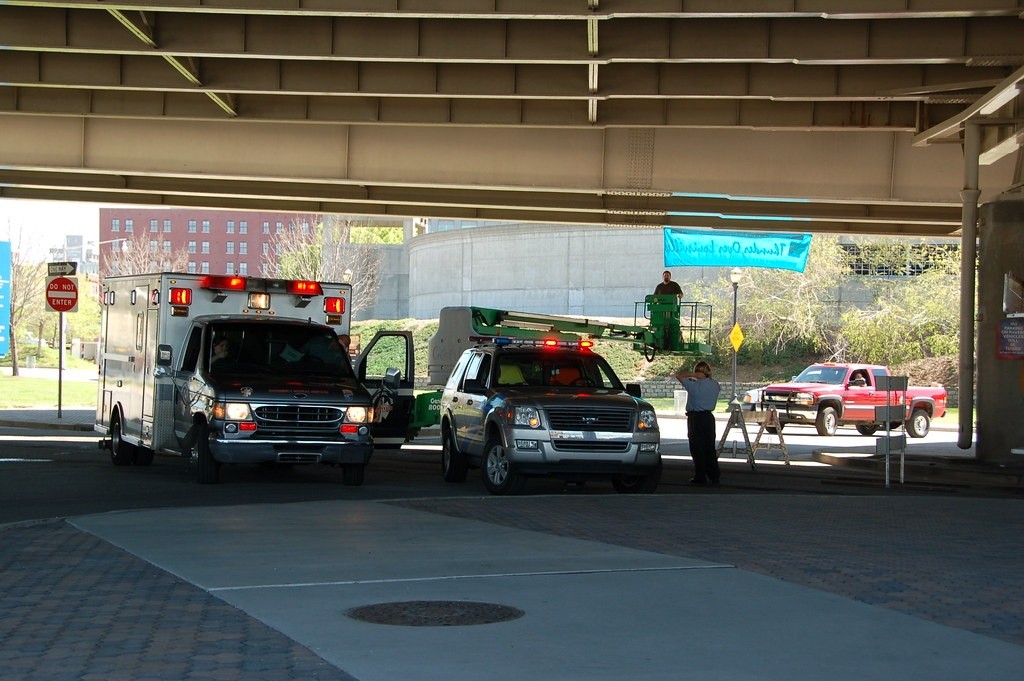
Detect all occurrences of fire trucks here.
[91,271,416,487]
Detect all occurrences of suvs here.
[438,334,663,498]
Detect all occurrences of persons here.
[676,361,722,489]
[851,373,868,388]
[653,271,683,305]
[208,335,234,374]
[297,334,352,369]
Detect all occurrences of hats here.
[338,334,351,347]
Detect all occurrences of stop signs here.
[45,275,79,312]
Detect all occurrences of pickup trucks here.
[759,361,948,439]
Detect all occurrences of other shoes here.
[713,480,720,486]
[689,477,707,484]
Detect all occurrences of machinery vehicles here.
[352,292,713,450]
